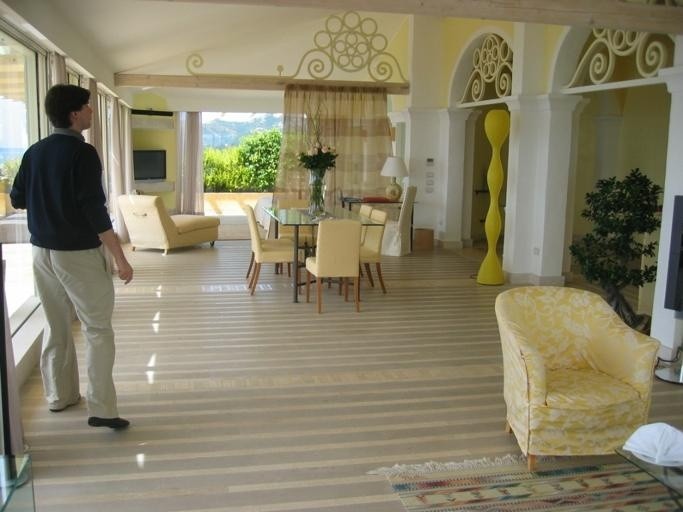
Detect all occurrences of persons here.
[9,83,132,429]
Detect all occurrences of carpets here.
[364,453,681,512]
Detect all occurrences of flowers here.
[293,88,340,215]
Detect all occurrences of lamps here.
[380,155,408,201]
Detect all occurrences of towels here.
[622,421,683,467]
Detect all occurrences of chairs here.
[118,193,221,254]
[238,185,418,315]
[491,284,661,475]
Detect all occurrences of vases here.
[308,170,327,216]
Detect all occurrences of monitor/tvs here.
[133,149,167,182]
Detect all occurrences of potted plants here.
[565,165,664,337]
